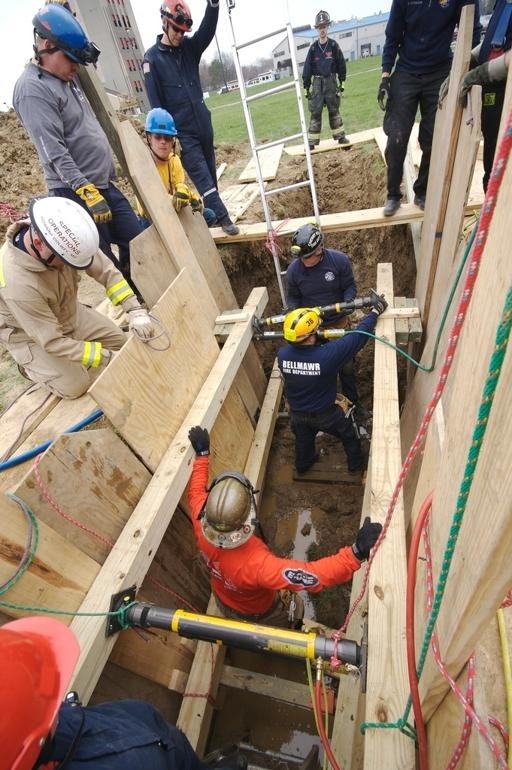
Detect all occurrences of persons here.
[438,1,511,196]
[302,11,350,153]
[2,616,204,768]
[282,223,360,407]
[188,426,381,627]
[147,108,204,216]
[13,4,143,295]
[0,196,154,400]
[378,0,483,217]
[276,294,388,476]
[141,0,240,237]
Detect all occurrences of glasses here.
[154,134,174,142]
[171,24,185,34]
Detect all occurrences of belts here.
[315,74,328,79]
[294,407,336,417]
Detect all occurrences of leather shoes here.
[414,194,426,210]
[298,453,319,474]
[222,223,239,236]
[304,145,314,151]
[349,450,368,471]
[339,138,350,143]
[383,200,401,216]
[350,400,370,418]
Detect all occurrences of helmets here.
[284,306,324,343]
[314,11,332,27]
[32,2,101,69]
[1,616,81,770]
[205,470,254,532]
[144,107,178,136]
[160,0,193,33]
[29,194,100,269]
[290,223,324,259]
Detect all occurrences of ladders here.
[231,22,323,311]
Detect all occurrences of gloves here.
[171,192,189,213]
[439,55,479,103]
[211,0,220,4]
[75,182,113,223]
[172,137,182,158]
[352,517,382,560]
[372,294,388,316]
[282,304,295,315]
[304,88,311,99]
[127,307,154,343]
[189,197,203,214]
[188,425,210,456]
[338,80,345,92]
[377,77,390,111]
[101,348,119,366]
[456,62,488,109]
